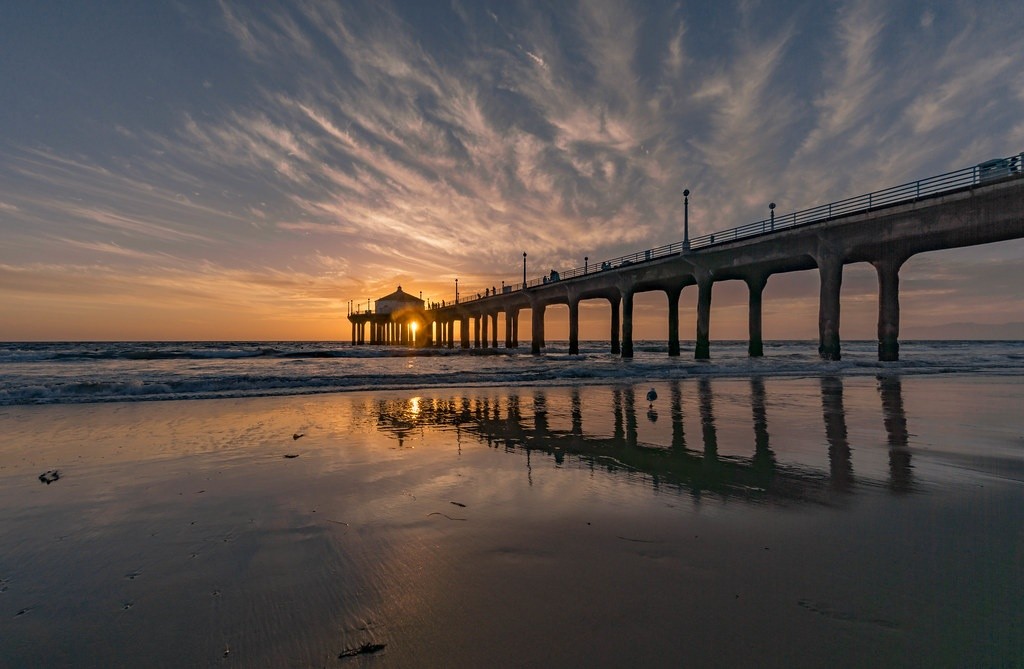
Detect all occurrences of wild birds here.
[646,388,657,405]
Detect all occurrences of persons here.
[543,270,559,283]
[491,286,496,295]
[486,288,489,296]
[477,294,481,298]
[432,300,445,309]
[601,262,611,270]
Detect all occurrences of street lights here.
[427,298,429,310]
[420,291,423,300]
[502,280,504,287]
[348,301,350,316]
[683,188,690,250]
[522,251,527,289]
[584,256,588,275]
[367,298,370,310]
[769,202,777,233]
[455,279,458,305]
[351,300,353,316]
[358,304,360,313]
